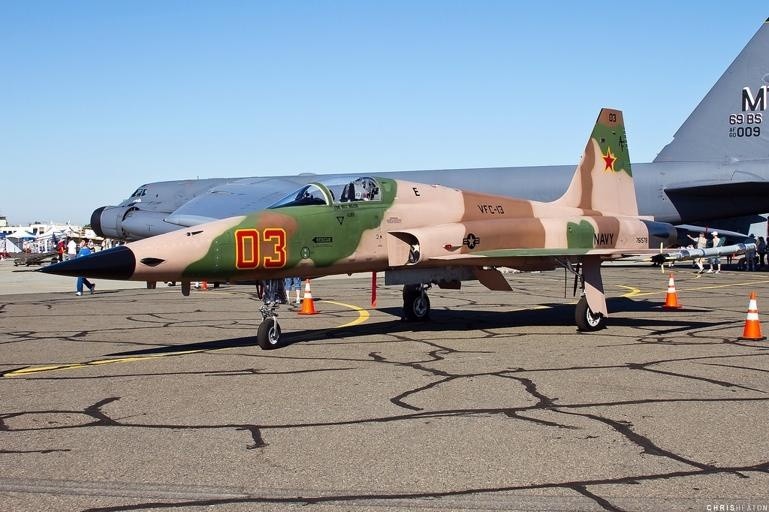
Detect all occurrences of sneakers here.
[91,284,95,294]
[286,298,300,305]
[75,292,83,296]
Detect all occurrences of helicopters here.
[8,240,63,268]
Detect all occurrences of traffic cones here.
[200,280,211,292]
[295,277,322,317]
[738,288,767,342]
[659,269,685,310]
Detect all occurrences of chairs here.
[340,183,355,202]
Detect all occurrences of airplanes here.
[87,12,769,316]
[12,105,693,349]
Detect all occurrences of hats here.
[711,231,718,235]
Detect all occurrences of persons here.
[283,277,301,304]
[100,239,105,251]
[57,239,65,262]
[74,238,95,296]
[65,238,76,261]
[685,230,768,274]
